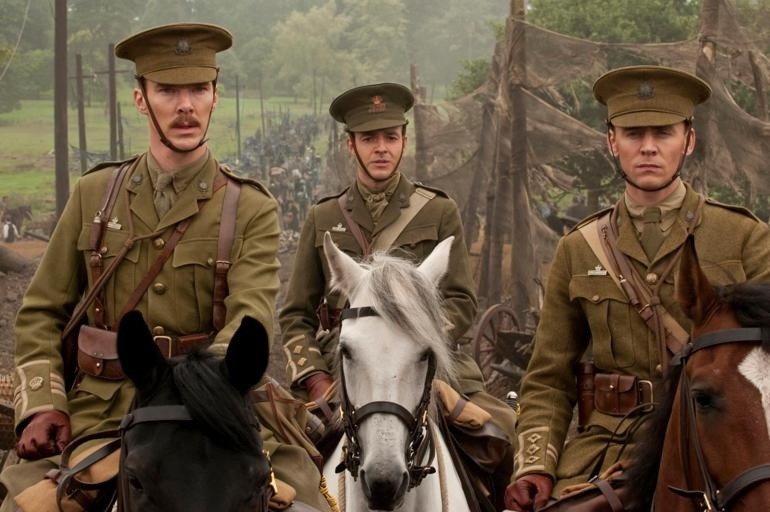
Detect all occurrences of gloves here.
[304,373,334,402]
[16,410,72,460]
[503,473,553,512]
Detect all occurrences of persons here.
[216,107,344,257]
[281,82,518,512]
[0,195,10,220]
[3,215,21,243]
[0,21,340,512]
[504,65,770,512]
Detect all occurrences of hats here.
[115,23,233,85]
[328,83,414,133]
[592,65,713,129]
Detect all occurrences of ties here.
[152,173,179,221]
[365,192,389,228]
[639,207,665,263]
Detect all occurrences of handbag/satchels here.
[75,324,127,381]
[595,373,639,418]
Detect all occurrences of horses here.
[542,233,770,511]
[116,309,270,511]
[2,205,33,234]
[323,230,471,511]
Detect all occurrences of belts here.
[635,379,666,413]
[150,333,214,360]
